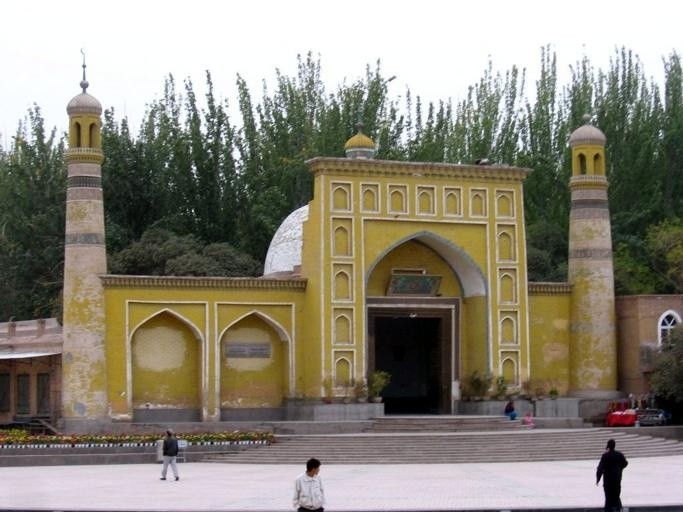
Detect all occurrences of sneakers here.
[160,477,179,480]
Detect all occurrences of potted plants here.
[460,370,559,401]
[321,372,391,403]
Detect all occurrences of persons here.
[521,411,535,429]
[290,458,325,512]
[595,439,627,511]
[504,401,518,420]
[157,428,179,481]
